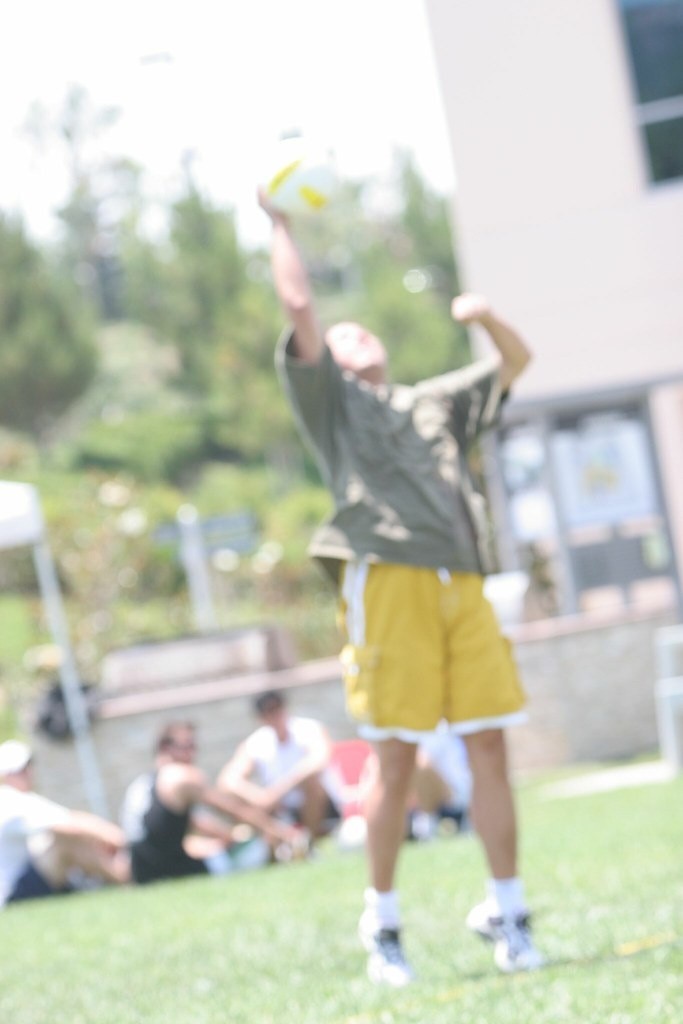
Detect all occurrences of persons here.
[404,720,473,840]
[0,723,311,902]
[253,182,543,985]
[214,690,338,858]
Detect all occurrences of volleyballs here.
[258,136,342,220]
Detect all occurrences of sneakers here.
[468,904,541,971]
[355,913,414,987]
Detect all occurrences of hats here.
[0,740,32,775]
